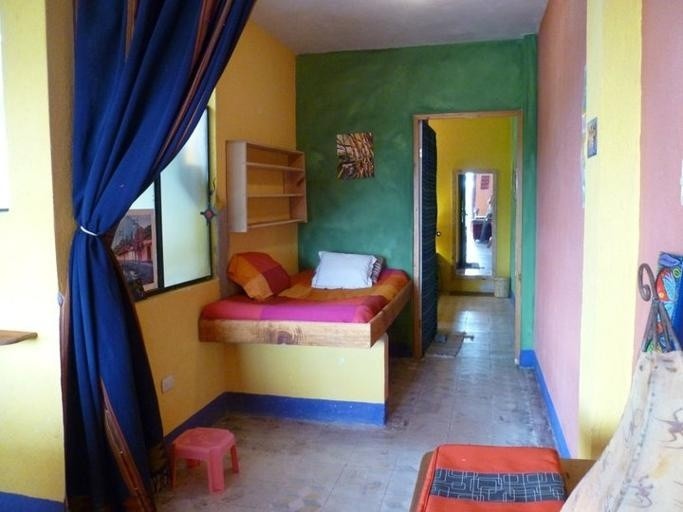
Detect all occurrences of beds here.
[198,268,413,349]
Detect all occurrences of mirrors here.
[452,168,499,280]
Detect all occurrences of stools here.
[495,277,511,298]
[169,427,240,495]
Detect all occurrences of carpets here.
[427,328,466,358]
[410,450,597,511]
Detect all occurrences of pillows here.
[310,250,384,290]
[226,253,291,301]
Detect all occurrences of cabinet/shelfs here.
[226,140,309,234]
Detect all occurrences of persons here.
[475,191,493,244]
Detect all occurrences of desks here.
[473,219,491,225]
[0,330,37,346]
